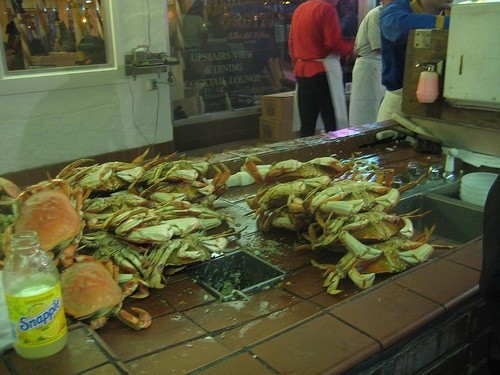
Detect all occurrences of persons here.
[380,0,454,123]
[289,0,356,138]
[348,0,399,126]
[175,7,209,81]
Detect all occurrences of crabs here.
[243,153,437,296]
[0,146,249,336]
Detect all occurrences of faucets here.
[431,148,460,183]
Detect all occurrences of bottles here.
[3,230,68,359]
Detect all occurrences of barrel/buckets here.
[460,172,498,205]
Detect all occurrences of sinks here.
[430,169,487,210]
[389,191,484,255]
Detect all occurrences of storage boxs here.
[259,91,321,144]
